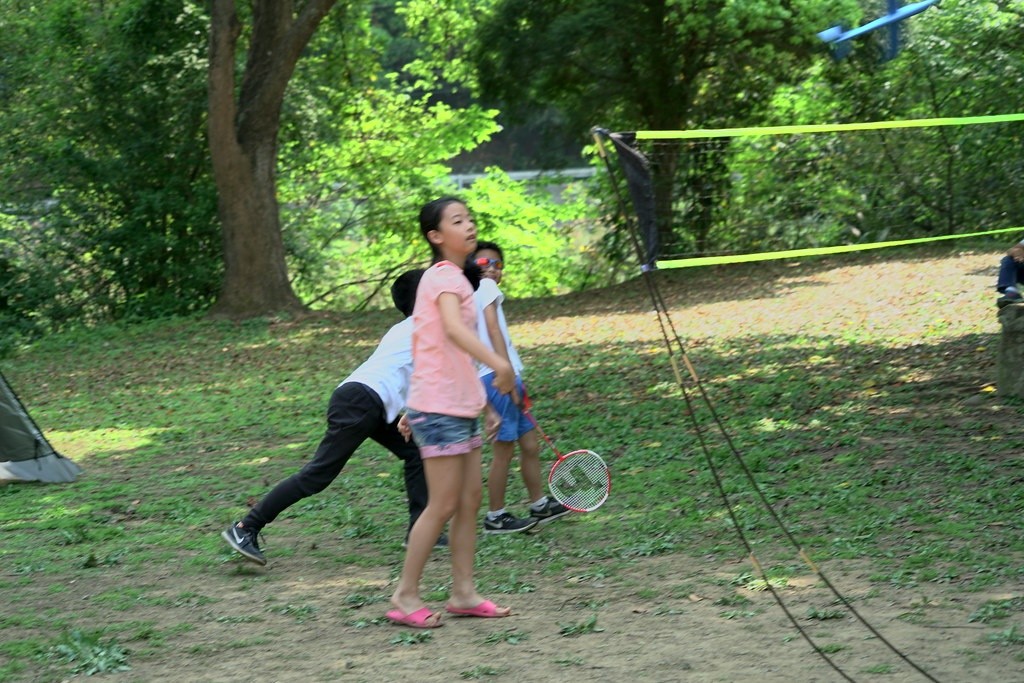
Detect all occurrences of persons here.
[995,238,1024,309]
[221,195,572,628]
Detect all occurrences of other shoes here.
[997,291,1024,308]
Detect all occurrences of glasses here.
[474,257,504,270]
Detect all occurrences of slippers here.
[385,607,444,629]
[445,599,511,617]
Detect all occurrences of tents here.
[0,372,83,484]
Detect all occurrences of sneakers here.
[401,532,448,547]
[484,512,534,533]
[529,497,572,527]
[221,521,268,566]
